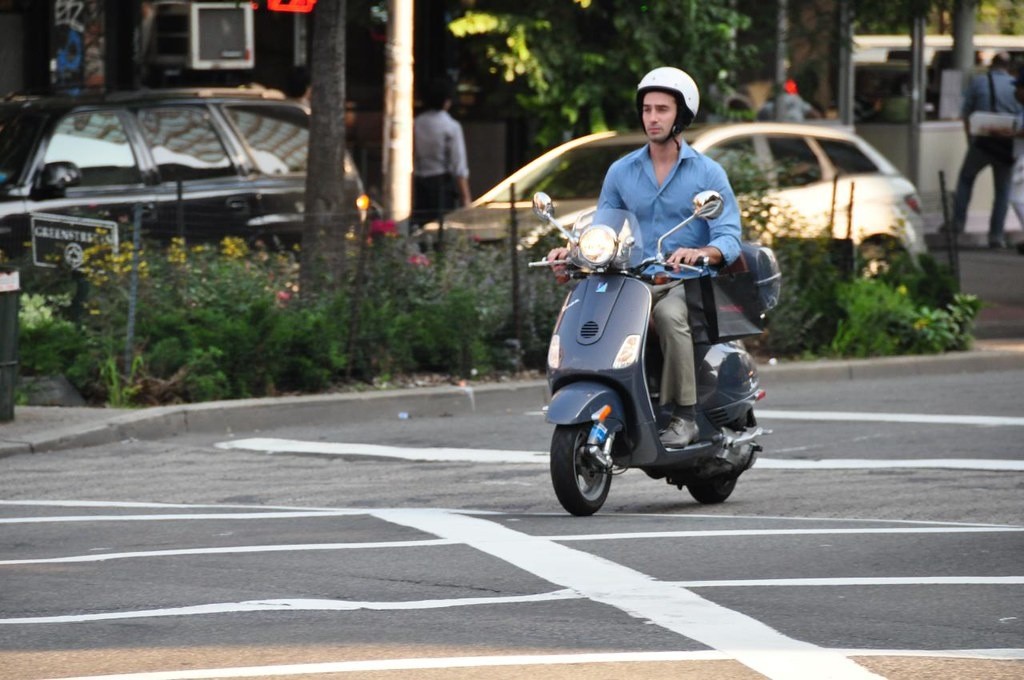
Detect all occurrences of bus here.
[849,34,1024,250]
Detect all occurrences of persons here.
[938,52,1024,250]
[287,65,472,228]
[547,67,743,449]
[757,74,911,125]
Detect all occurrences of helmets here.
[635,67,700,126]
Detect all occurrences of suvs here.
[0,87,370,259]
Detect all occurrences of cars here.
[414,121,927,282]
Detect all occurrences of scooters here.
[529,192,782,515]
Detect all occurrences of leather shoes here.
[659,418,701,448]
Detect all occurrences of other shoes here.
[939,221,963,233]
[989,233,1007,249]
